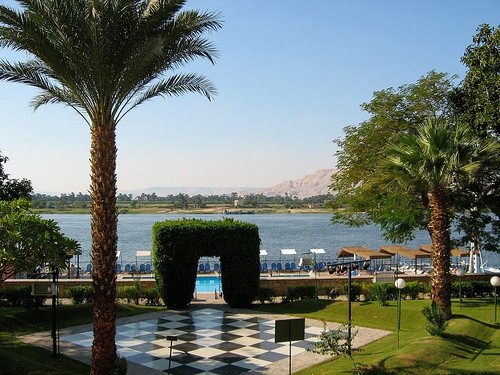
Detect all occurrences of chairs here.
[29,240,486,296]
